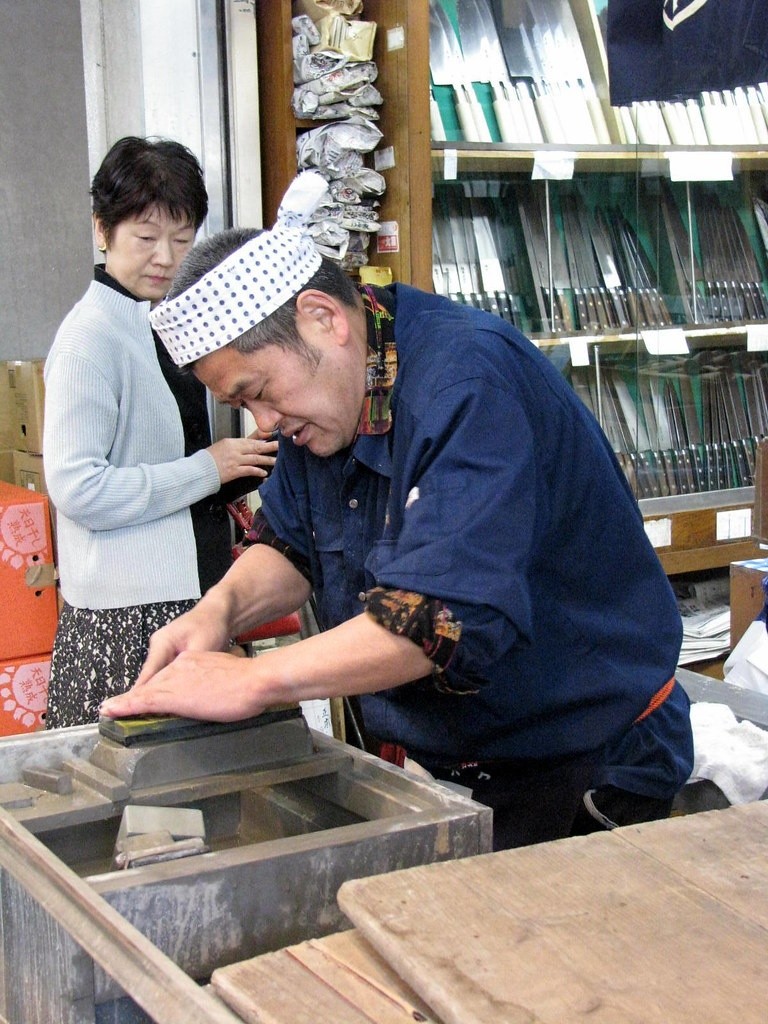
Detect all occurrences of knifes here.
[427,0,768,503]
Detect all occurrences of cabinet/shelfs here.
[257,0,768,674]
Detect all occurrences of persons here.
[44,138,279,727]
[95,228,693,850]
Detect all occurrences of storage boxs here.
[0,357,64,734]
